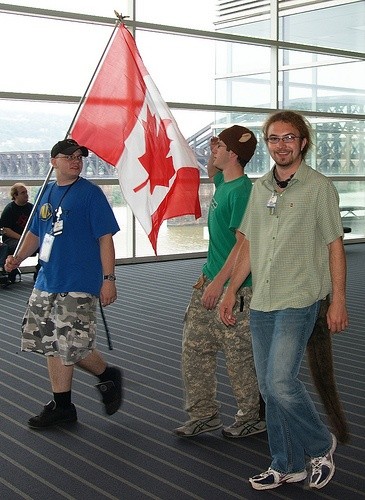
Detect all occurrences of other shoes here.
[8,273,16,283]
[34,266,40,282]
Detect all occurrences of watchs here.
[104,273,116,281]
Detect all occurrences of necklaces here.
[273,165,296,189]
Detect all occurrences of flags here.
[71,20,202,257]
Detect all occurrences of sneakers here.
[28,400,77,430]
[249,467,307,490]
[96,367,122,415]
[176,413,223,436]
[309,433,338,490]
[222,417,267,437]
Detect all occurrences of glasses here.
[55,155,83,161]
[264,136,303,144]
[217,142,227,149]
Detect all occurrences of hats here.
[218,125,257,162]
[51,139,88,158]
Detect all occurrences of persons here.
[0,183,41,286]
[219,112,348,493]
[173,125,267,439]
[5,139,124,429]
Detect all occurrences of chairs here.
[0,230,41,284]
